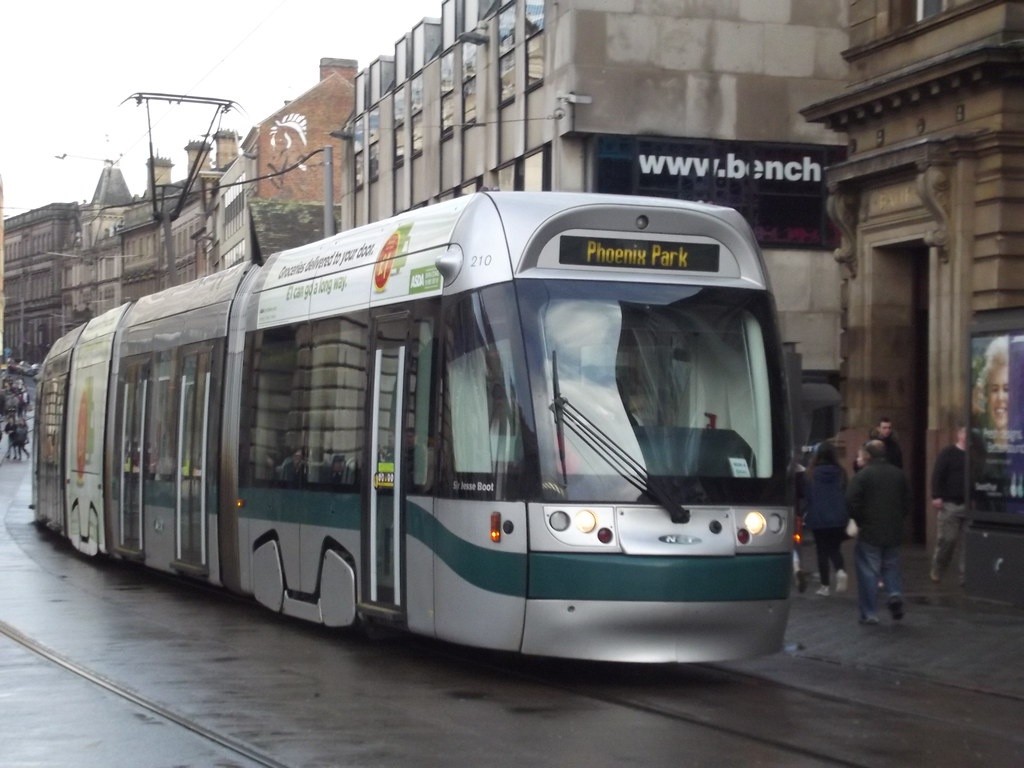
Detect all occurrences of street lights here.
[328,127,354,232]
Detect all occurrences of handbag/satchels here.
[24,438,29,444]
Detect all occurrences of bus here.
[32,92,806,670]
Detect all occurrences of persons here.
[800,443,847,596]
[854,419,902,470]
[847,441,908,623]
[0,375,31,462]
[795,453,807,593]
[930,421,967,584]
[972,337,1009,453]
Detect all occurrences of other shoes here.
[815,585,830,596]
[929,568,941,582]
[835,569,848,592]
[793,570,810,592]
[858,616,878,624]
[886,592,905,620]
